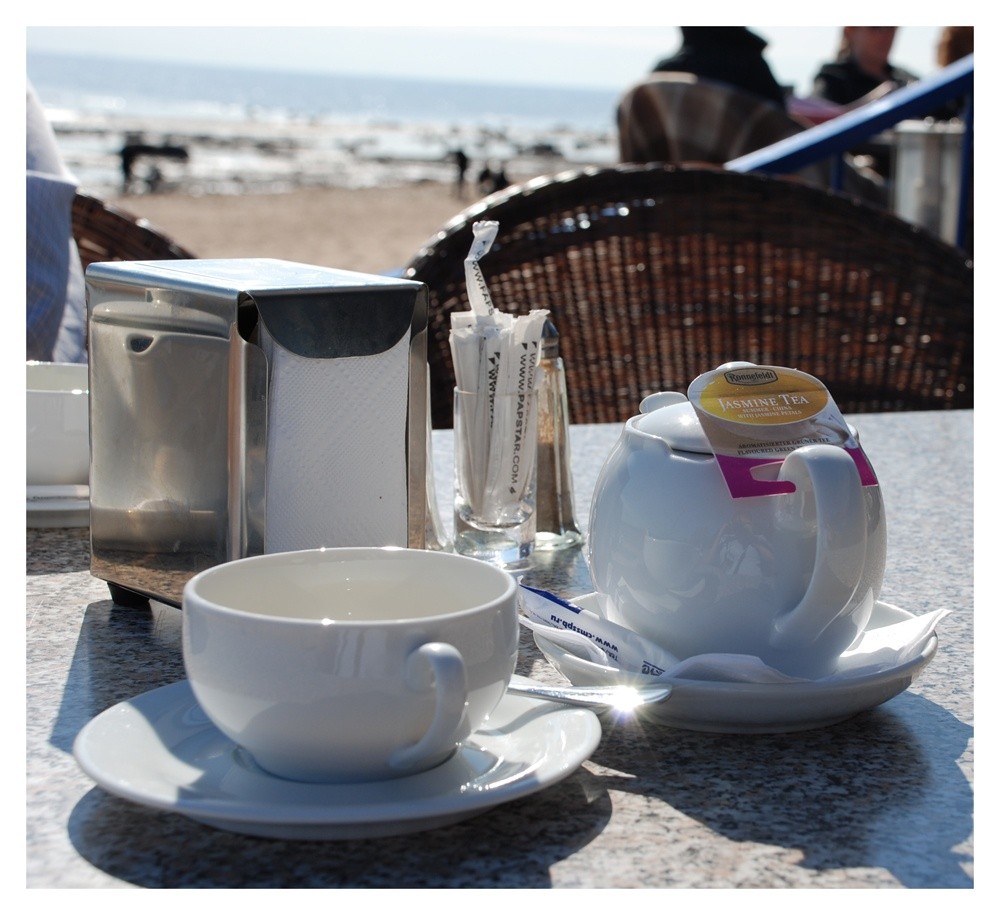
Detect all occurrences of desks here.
[27,405,974,889]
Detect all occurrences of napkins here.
[254,316,412,554]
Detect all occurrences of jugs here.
[587,361,886,678]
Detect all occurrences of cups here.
[26,360,89,485]
[688,366,849,445]
[450,385,542,579]
[182,547,516,782]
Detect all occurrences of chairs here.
[72,68,974,433]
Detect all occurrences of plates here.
[532,590,939,733]
[72,672,601,838]
[26,485,90,529]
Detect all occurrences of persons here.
[26,75,88,366]
[645,26,975,186]
[450,145,512,197]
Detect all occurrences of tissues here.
[74,257,427,607]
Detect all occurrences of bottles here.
[532,319,584,552]
